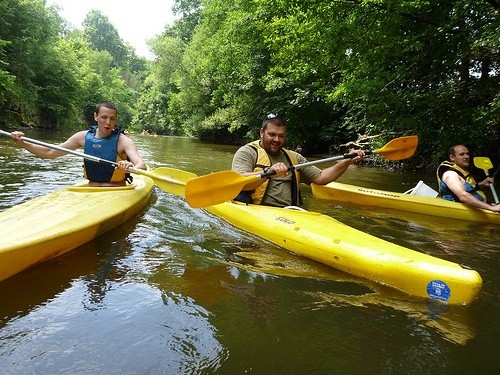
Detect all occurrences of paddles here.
[185,134,419,209]
[473,156,500,205]
[0,130,199,199]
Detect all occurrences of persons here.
[143,130,149,135]
[437,145,500,211]
[11,103,147,186]
[232,114,365,209]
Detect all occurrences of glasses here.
[264,113,278,122]
